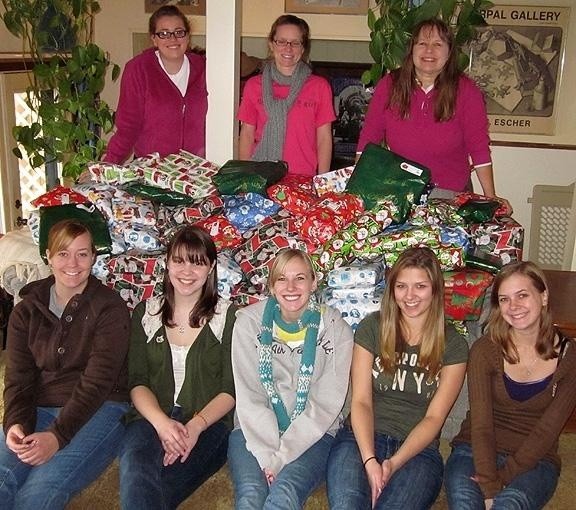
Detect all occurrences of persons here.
[0,219,130,510]
[445,261,576,510]
[228,249,354,510]
[102,6,208,164]
[236,15,337,176]
[356,18,513,217]
[326,248,469,510]
[120,226,238,510]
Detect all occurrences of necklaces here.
[171,312,189,336]
[510,327,542,377]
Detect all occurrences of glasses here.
[155,29,188,39]
[272,38,305,47]
